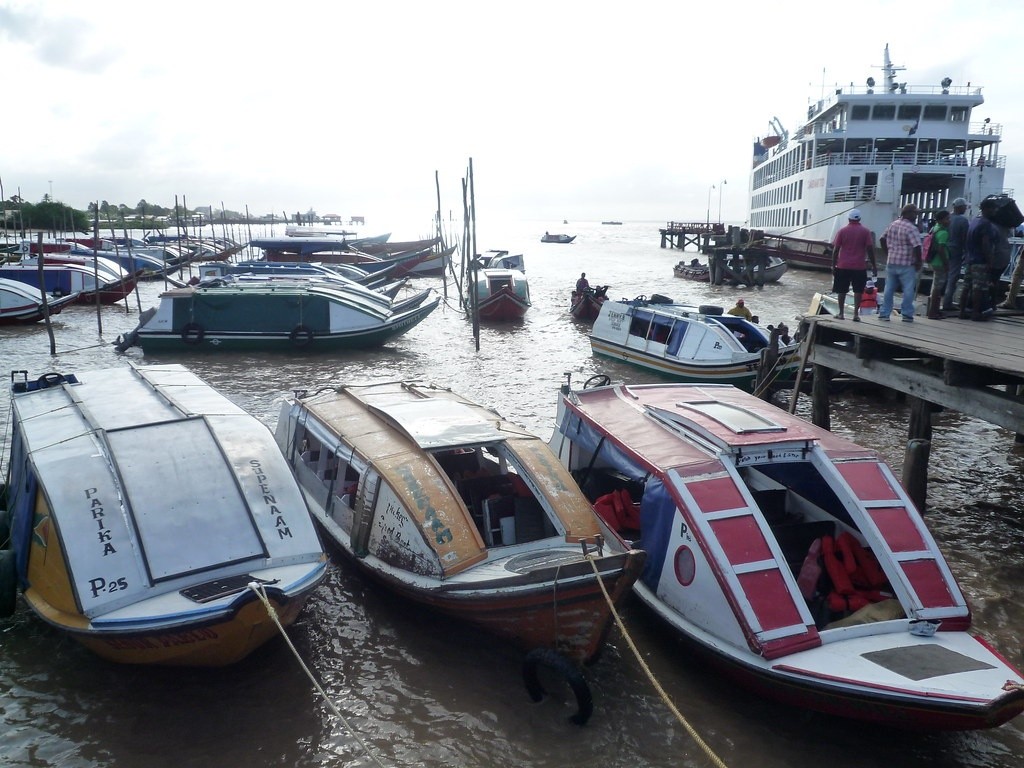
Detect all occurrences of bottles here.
[871,271,878,282]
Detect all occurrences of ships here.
[748,42,1015,269]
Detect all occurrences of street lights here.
[706,185,716,224]
[720,180,728,224]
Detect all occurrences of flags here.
[909,118,919,135]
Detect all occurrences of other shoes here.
[996,300,1015,310]
[971,313,991,322]
[959,312,972,319]
[853,316,860,321]
[901,315,913,322]
[833,314,844,320]
[943,305,960,311]
[878,316,890,321]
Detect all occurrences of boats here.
[568,287,602,319]
[0,229,460,359]
[563,220,568,224]
[540,230,578,243]
[542,371,1024,733]
[463,250,533,320]
[673,262,711,282]
[726,256,788,284]
[761,135,780,148]
[272,375,647,666]
[601,220,624,226]
[5,361,332,672]
[584,294,803,384]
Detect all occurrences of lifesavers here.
[698,305,724,315]
[651,293,674,304]
[522,644,594,727]
[289,324,312,351]
[1,483,20,616]
[180,321,205,345]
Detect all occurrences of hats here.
[866,281,875,288]
[738,299,744,304]
[953,198,972,207]
[903,203,922,212]
[848,208,861,221]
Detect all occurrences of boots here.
[927,297,947,319]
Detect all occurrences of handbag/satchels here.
[981,195,1024,228]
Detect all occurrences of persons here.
[958,209,1011,322]
[767,322,793,346]
[942,198,969,311]
[878,204,923,322]
[859,281,881,315]
[727,299,752,322]
[576,272,589,303]
[571,291,578,305]
[996,248,1024,309]
[594,286,608,303]
[833,209,877,322]
[752,316,759,324]
[926,211,950,320]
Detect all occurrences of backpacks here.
[921,227,949,263]
